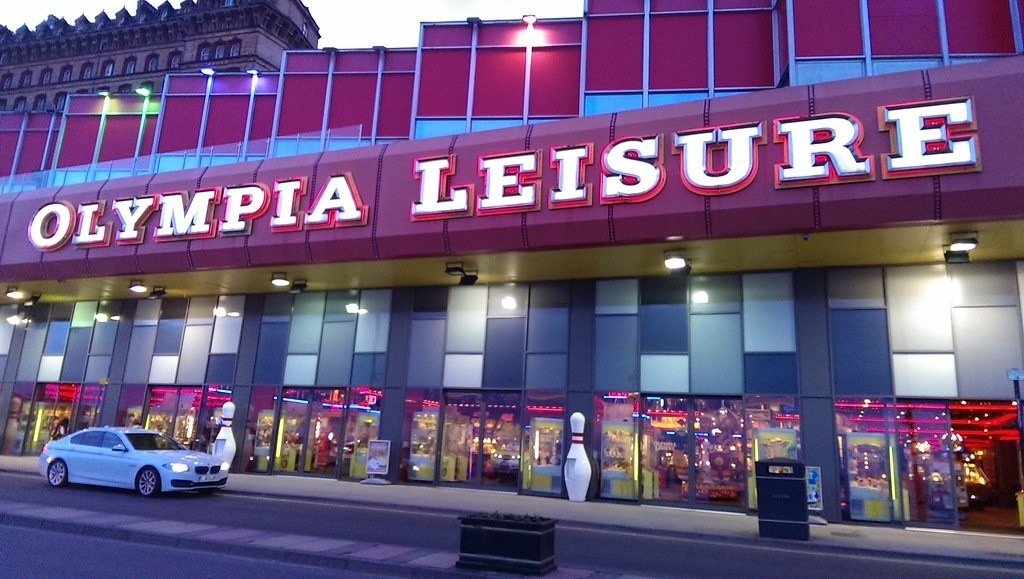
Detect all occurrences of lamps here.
[670,260,691,277]
[129,279,147,293]
[291,280,306,294]
[24,293,42,306]
[149,286,166,300]
[944,246,970,263]
[665,251,686,269]
[5,287,23,301]
[949,232,979,252]
[271,272,288,287]
[459,269,477,286]
[446,264,463,277]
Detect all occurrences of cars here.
[39,427,230,498]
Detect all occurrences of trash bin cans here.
[755,458,810,540]
